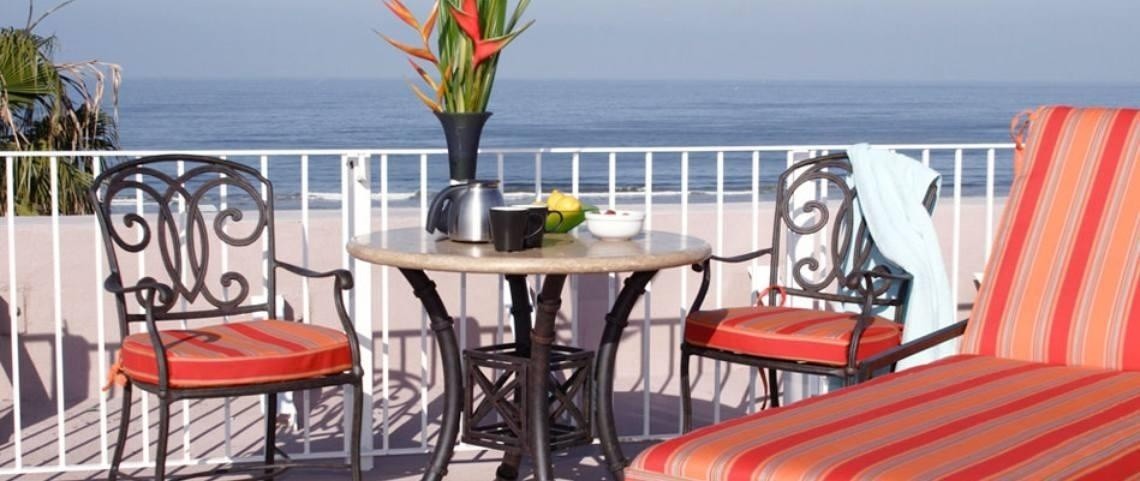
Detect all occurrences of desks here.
[344,220,712,481]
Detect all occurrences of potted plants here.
[368,0,538,234]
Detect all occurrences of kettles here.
[426,179,504,244]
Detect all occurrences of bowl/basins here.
[585,211,646,243]
[545,206,600,234]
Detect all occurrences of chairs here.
[88,152,367,480]
[620,101,1140,479]
[675,151,940,434]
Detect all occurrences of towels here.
[829,142,962,395]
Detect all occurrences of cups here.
[489,207,544,253]
[511,205,563,249]
